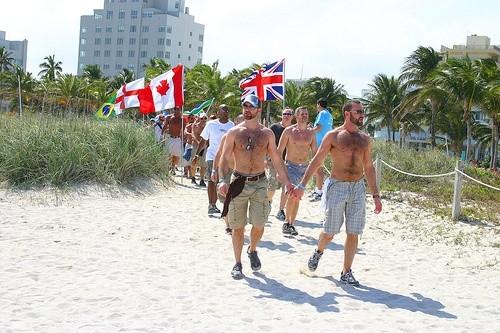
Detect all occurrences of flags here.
[137,64,184,115]
[92,93,119,122]
[181,97,214,117]
[112,76,145,117]
[238,60,286,103]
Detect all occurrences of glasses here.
[246,136,253,150]
[201,117,207,119]
[221,109,229,113]
[350,109,366,113]
[160,119,164,121]
[282,112,293,115]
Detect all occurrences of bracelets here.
[312,126,314,132]
[218,178,227,183]
[297,182,306,189]
[211,169,218,173]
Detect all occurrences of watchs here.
[372,194,380,198]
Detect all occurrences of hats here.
[199,113,207,118]
[241,93,261,107]
[317,96,328,102]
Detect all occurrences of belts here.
[233,172,266,181]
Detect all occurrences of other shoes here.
[200,180,206,188]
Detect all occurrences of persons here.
[265,105,295,221]
[290,100,383,288]
[210,113,259,236]
[306,96,334,202]
[217,94,300,279]
[274,104,323,238]
[143,106,221,186]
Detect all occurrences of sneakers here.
[225,226,232,235]
[231,262,243,277]
[308,191,324,202]
[308,248,324,271]
[165,164,197,185]
[277,209,285,220]
[247,246,261,270]
[340,269,360,285]
[282,222,298,236]
[208,204,222,215]
